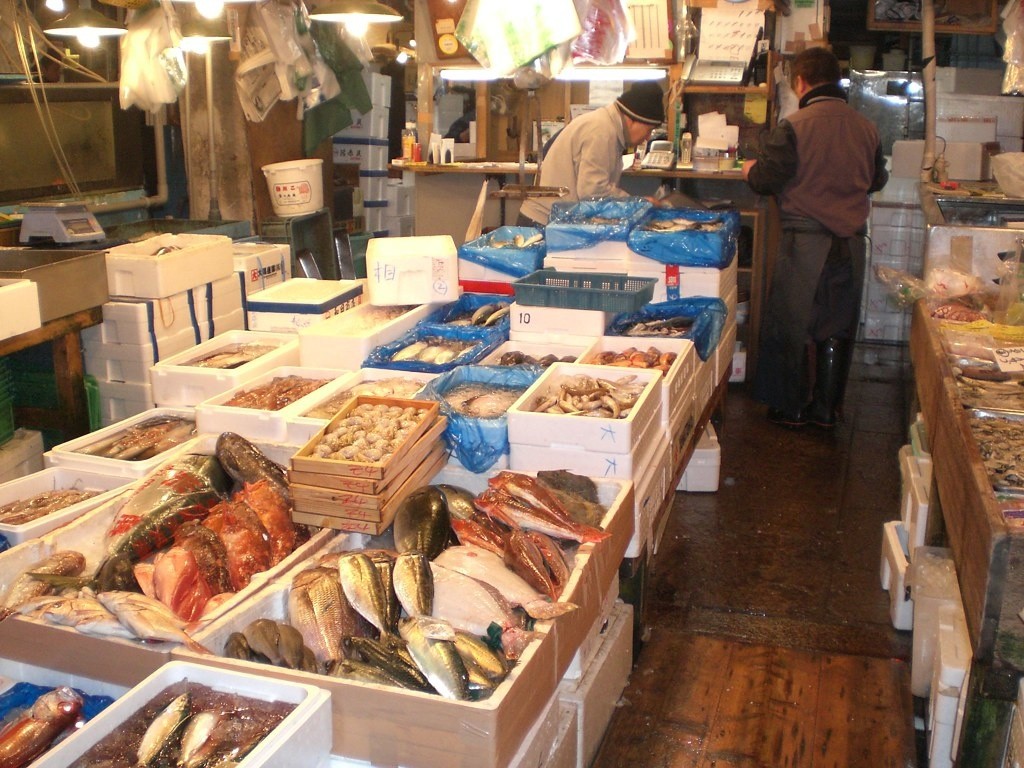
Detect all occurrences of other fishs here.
[0,217,735,768]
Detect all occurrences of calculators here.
[642,140,675,168]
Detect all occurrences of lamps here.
[181,14,232,54]
[43,0,128,48]
[308,0,405,38]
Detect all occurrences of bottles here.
[402,122,418,159]
[932,154,945,183]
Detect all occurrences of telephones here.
[677,132,694,169]
[680,54,747,84]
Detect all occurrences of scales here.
[17,198,103,245]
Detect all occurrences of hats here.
[616,81,665,126]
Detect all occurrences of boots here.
[767,337,854,431]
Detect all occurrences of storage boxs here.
[0,72,747,768]
[858,64,1024,768]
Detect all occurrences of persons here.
[443,116,472,143]
[516,80,665,227]
[742,48,890,426]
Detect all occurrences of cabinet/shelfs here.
[384,0,778,181]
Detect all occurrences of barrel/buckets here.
[261,159,323,217]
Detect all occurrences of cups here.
[719,152,734,170]
[939,171,948,182]
